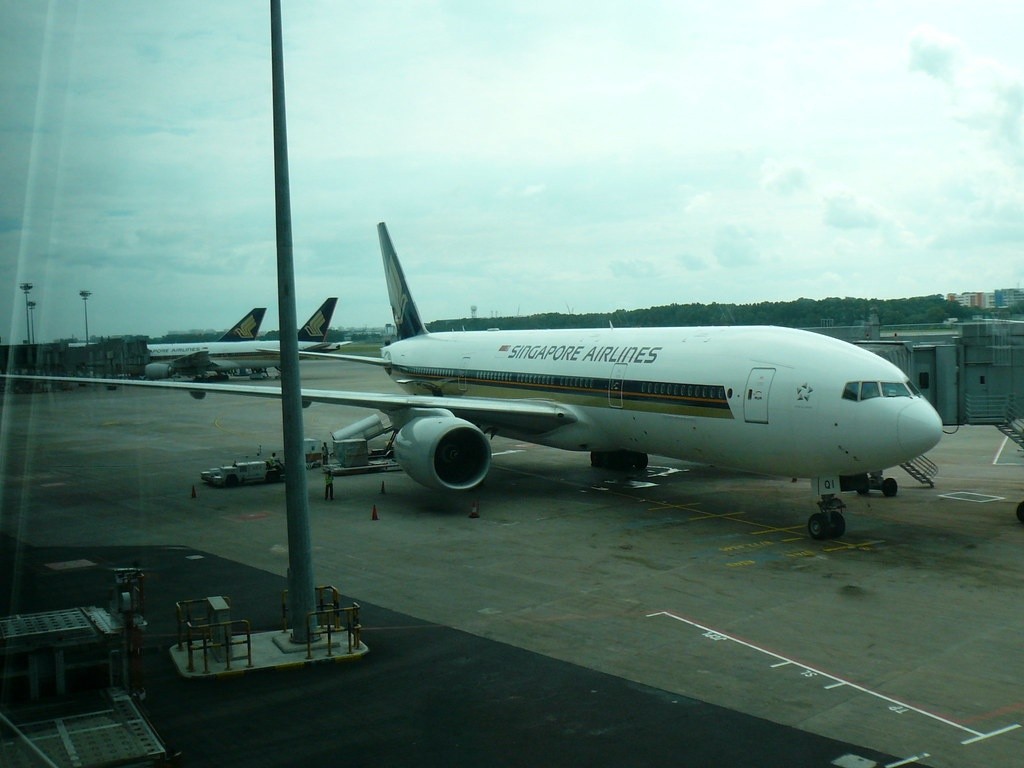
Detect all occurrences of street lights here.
[19,281,34,342]
[79,289,92,343]
[27,300,36,343]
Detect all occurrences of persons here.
[270,452,283,470]
[322,442,329,466]
[324,470,334,501]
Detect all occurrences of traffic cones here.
[379,480,386,495]
[468,500,480,518]
[370,504,379,520]
[191,486,197,498]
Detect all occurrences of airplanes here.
[69,296,354,383]
[1,221,946,540]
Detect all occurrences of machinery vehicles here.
[201,461,286,487]
